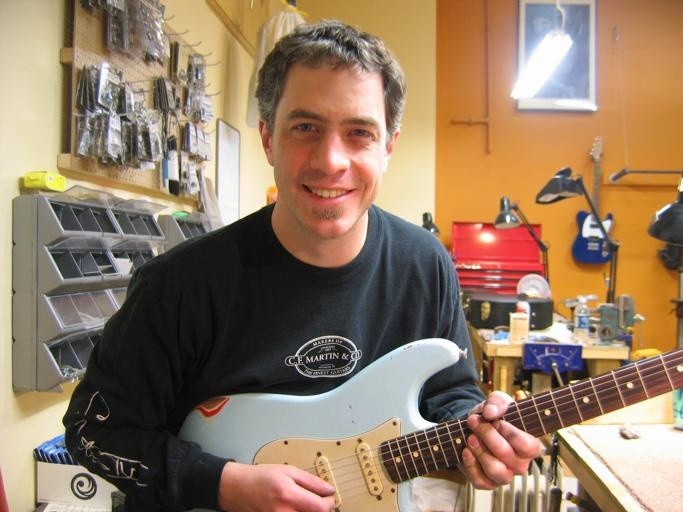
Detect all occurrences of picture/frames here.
[518,2,596,110]
[216,120,240,224]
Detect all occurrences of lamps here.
[535,167,620,302]
[510,0,575,100]
[648,176,683,351]
[422,212,440,236]
[494,197,549,289]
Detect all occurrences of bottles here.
[574,297,588,343]
[516,294,530,314]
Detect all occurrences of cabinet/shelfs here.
[12,196,165,392]
[158,215,210,252]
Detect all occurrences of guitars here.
[177,338,683,511]
[570,137,616,263]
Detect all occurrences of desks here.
[544,423,683,512]
[463,310,630,401]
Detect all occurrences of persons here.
[61,21,542,512]
